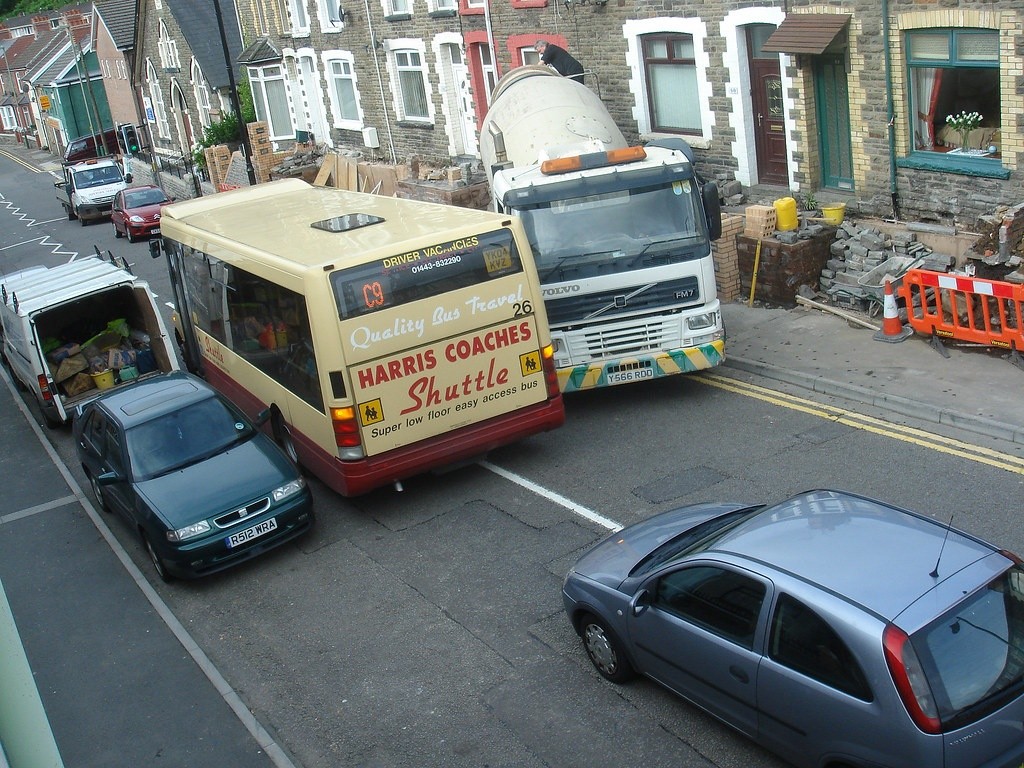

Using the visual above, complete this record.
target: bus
[149,176,567,514]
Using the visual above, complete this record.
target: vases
[960,130,971,152]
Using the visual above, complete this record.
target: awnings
[761,13,852,55]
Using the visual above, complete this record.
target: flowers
[946,111,983,151]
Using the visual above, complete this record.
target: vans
[63,126,119,163]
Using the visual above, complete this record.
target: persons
[534,40,584,86]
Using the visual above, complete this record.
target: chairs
[633,195,676,236]
[146,192,156,198]
[77,175,90,185]
[523,208,561,250]
[125,195,133,203]
[93,170,105,180]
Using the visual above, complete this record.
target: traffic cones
[872,281,914,344]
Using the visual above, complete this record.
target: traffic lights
[122,124,141,156]
[116,128,127,155]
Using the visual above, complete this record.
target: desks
[919,145,1002,159]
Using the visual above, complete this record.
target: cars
[109,184,177,243]
[70,368,319,587]
[560,485,1024,768]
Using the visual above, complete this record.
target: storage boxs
[48,343,81,364]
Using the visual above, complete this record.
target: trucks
[0,250,180,427]
[52,153,134,228]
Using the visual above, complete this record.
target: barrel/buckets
[821,202,846,225]
[90,369,114,390]
[773,197,798,232]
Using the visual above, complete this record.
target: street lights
[57,24,102,158]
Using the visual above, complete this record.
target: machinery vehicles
[475,63,727,393]
